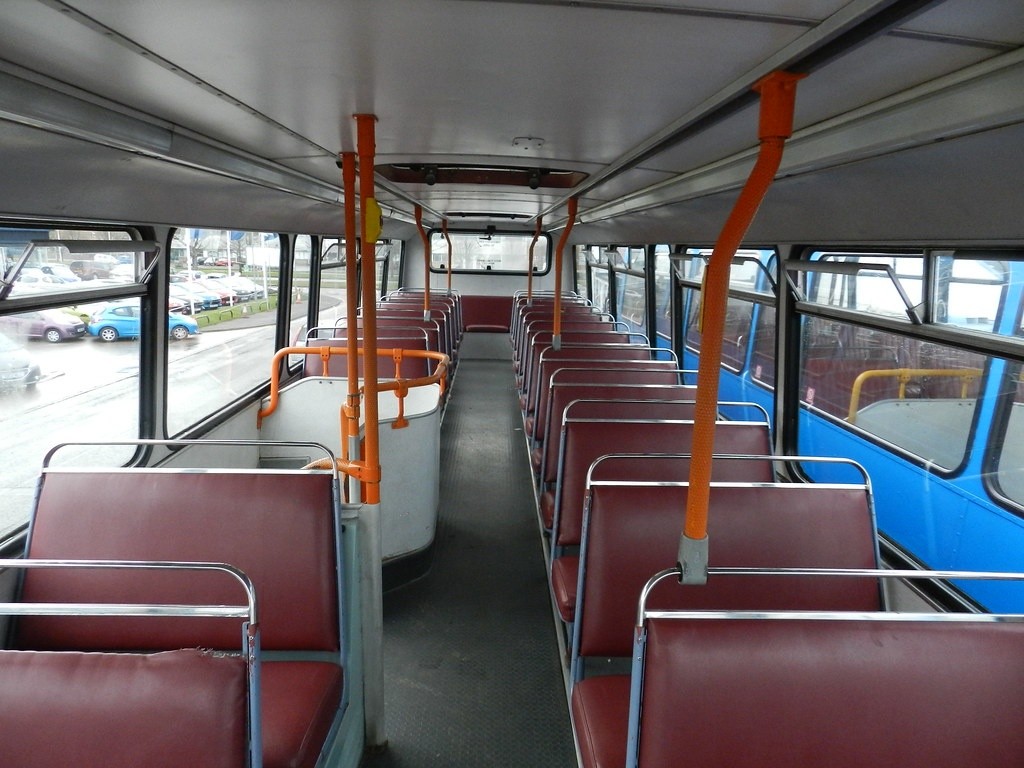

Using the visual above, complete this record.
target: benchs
[0,442,366,767]
[462,294,513,360]
[802,346,900,398]
[303,287,464,378]
[510,289,1024,768]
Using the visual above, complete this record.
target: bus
[594,240,1024,621]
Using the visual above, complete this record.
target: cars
[85,305,199,342]
[3,256,265,314]
[0,308,87,343]
